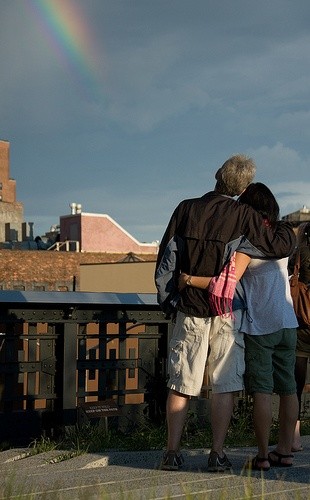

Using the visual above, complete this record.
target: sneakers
[161,450,185,470]
[208,449,233,471]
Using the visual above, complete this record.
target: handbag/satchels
[288,249,310,327]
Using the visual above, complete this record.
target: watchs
[185,275,192,286]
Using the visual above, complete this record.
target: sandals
[245,454,269,472]
[268,450,295,467]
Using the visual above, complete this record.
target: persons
[179,182,297,472]
[285,221,310,452]
[155,155,297,472]
